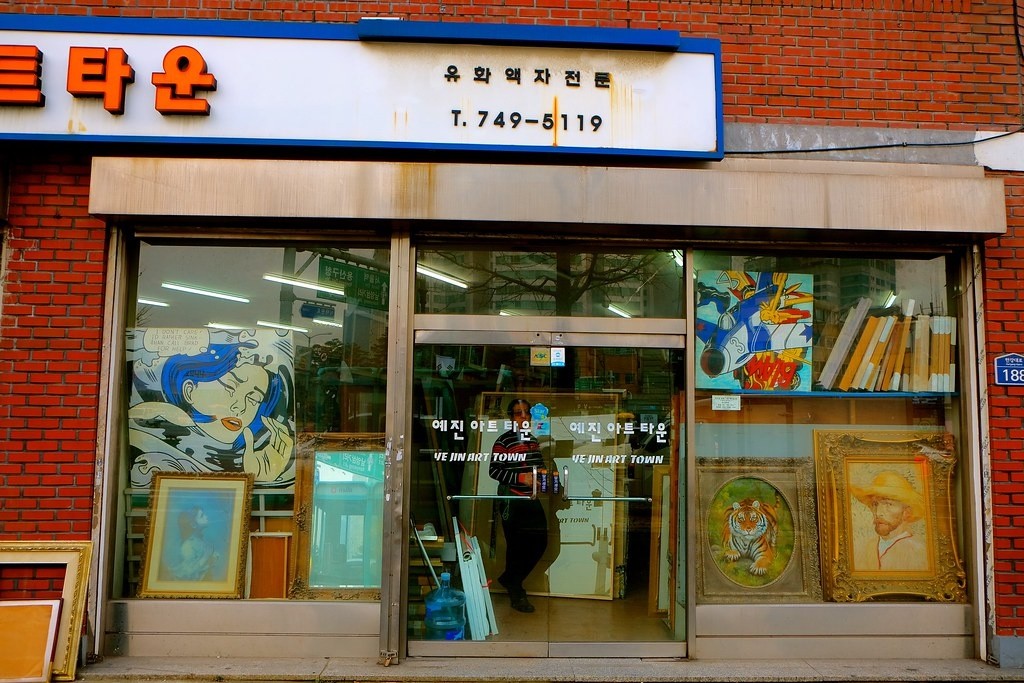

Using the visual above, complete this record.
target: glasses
[513,408,531,416]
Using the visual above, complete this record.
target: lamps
[262,271,345,297]
[137,296,170,307]
[256,320,308,333]
[160,280,250,303]
[668,250,698,279]
[416,263,468,290]
[602,301,632,318]
[203,323,254,330]
[312,318,342,327]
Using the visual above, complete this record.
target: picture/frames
[648,464,672,614]
[0,539,93,682]
[453,516,499,641]
[138,471,254,599]
[342,384,387,432]
[693,457,823,604]
[288,433,385,601]
[812,425,969,605]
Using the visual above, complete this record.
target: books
[813,297,958,393]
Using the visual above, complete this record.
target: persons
[488,399,550,613]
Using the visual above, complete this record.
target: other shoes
[499,576,524,596]
[510,595,535,613]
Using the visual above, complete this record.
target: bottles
[425,573,465,642]
